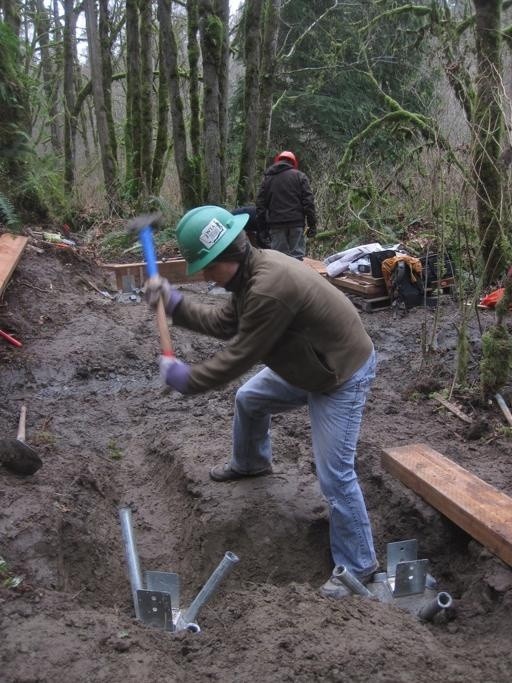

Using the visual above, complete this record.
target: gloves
[157,355,184,385]
[306,226,317,238]
[256,231,268,249]
[141,276,182,315]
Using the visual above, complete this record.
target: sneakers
[320,560,380,600]
[210,462,274,480]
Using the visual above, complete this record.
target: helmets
[175,206,251,277]
[274,151,297,168]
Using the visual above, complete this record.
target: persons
[135,202,381,601]
[260,146,318,261]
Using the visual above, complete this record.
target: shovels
[0,406,43,476]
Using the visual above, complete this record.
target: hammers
[128,214,173,357]
